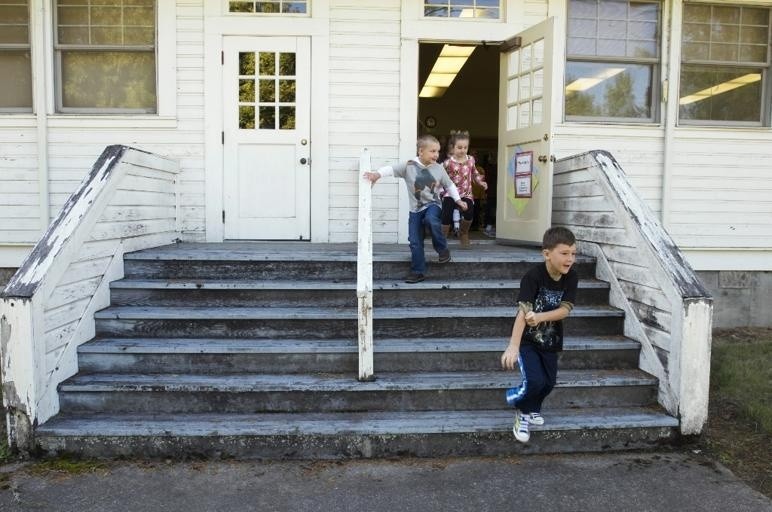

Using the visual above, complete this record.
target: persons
[500,227,579,444]
[440,130,489,249]
[363,136,468,283]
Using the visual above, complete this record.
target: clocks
[425,116,437,128]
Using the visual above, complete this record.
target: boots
[458,220,473,250]
[440,224,451,239]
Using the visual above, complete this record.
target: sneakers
[512,410,545,444]
[438,249,451,264]
[405,274,425,283]
